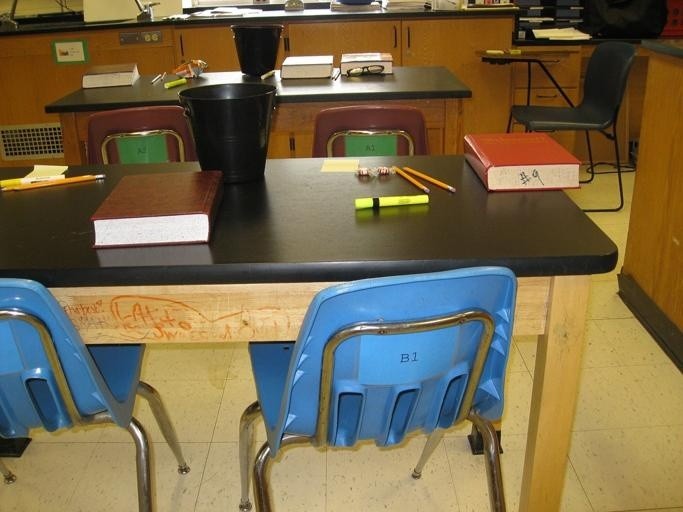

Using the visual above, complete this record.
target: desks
[46,66,474,164]
[1,155,619,512]
[475,44,580,65]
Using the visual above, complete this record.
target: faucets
[133,0,161,21]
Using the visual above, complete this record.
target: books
[532,27,593,41]
[329,1,381,13]
[463,133,582,194]
[91,170,221,247]
[341,52,393,76]
[281,55,335,78]
[193,7,264,17]
[386,0,425,13]
[81,64,141,89]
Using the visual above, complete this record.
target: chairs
[85,106,197,164]
[239,265,518,512]
[311,106,430,158]
[1,279,191,512]
[506,40,639,212]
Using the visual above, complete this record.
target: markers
[164,78,187,89]
[353,195,432,210]
[261,70,275,80]
[0,174,65,187]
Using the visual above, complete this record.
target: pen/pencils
[332,71,340,80]
[0,174,106,192]
[335,72,342,80]
[151,74,161,84]
[160,72,167,81]
[392,164,430,194]
[403,166,456,194]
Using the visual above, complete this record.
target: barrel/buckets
[176,83,279,186]
[231,24,284,78]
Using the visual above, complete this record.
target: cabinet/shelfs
[174,15,519,163]
[509,34,578,154]
